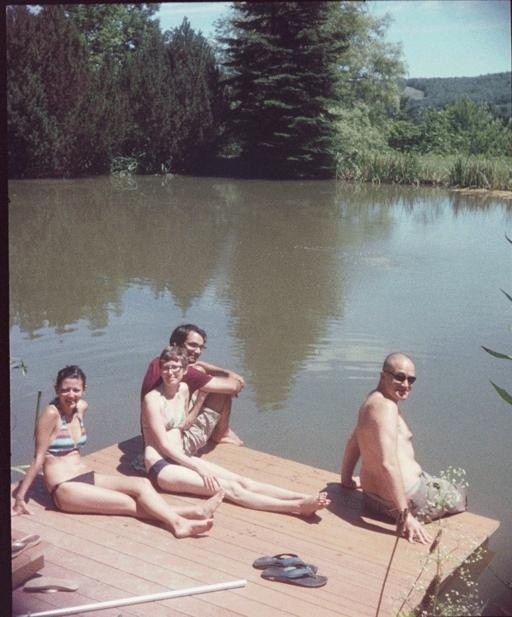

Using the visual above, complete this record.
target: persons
[140,347,333,519]
[11,365,225,540]
[341,352,468,545]
[138,324,245,447]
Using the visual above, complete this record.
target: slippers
[23,576,80,594]
[253,553,328,588]
[11,533,41,559]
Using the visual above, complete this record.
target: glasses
[384,370,416,385]
[160,365,184,373]
